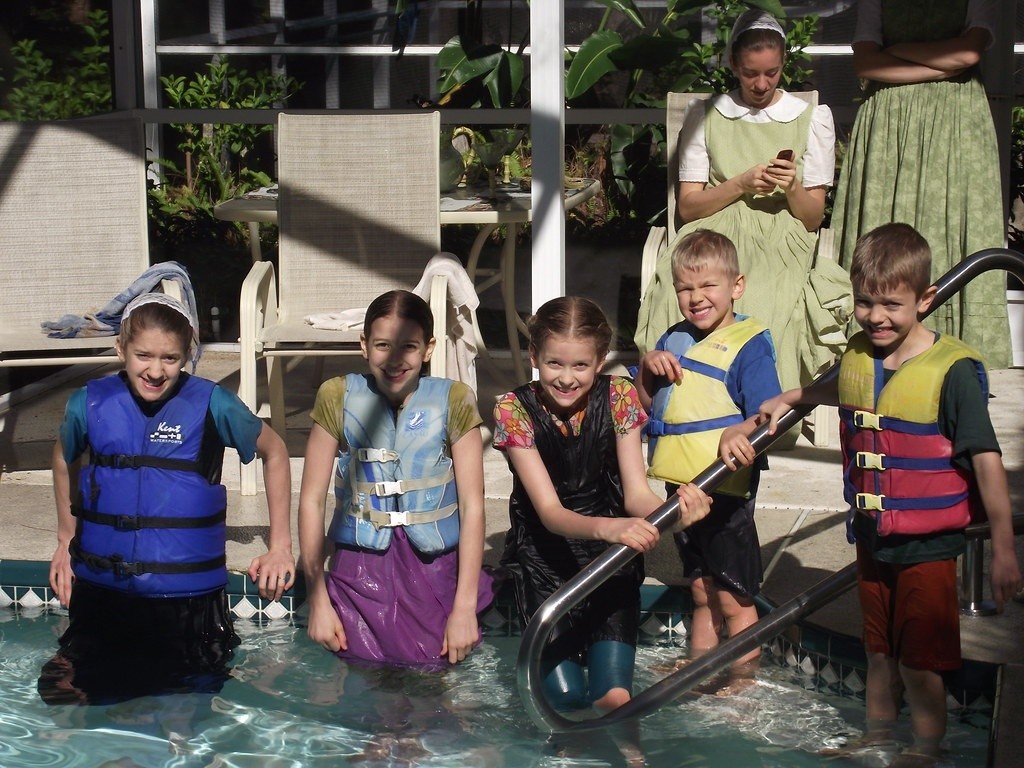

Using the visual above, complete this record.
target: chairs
[0,118,195,377]
[639,89,836,447]
[238,111,449,497]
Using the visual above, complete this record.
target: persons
[298,291,507,665]
[759,223,1022,737]
[633,10,835,450]
[493,297,713,717]
[50,293,296,703]
[830,1,1013,369]
[635,230,783,650]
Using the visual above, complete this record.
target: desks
[213,176,602,395]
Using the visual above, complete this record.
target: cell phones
[774,149,793,168]
